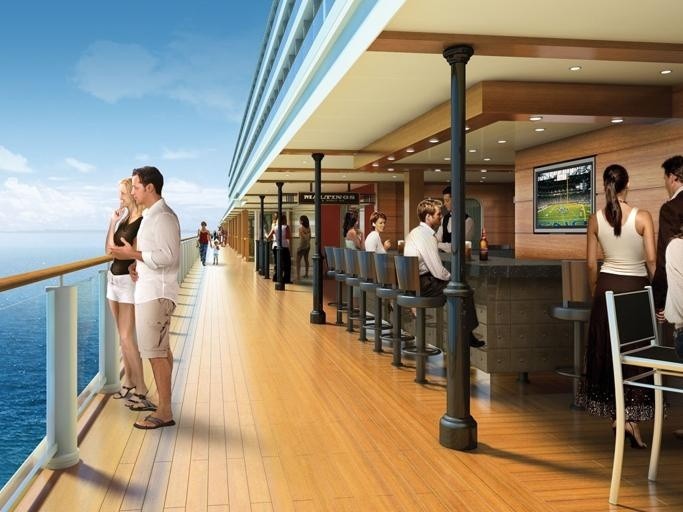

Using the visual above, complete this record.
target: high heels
[621,414,650,451]
[111,384,149,406]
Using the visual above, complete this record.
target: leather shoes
[467,331,486,350]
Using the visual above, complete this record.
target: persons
[209,241,219,266]
[268,215,292,284]
[209,227,228,248]
[196,220,211,266]
[402,198,486,348]
[582,164,669,450]
[649,155,683,325]
[295,214,311,280]
[341,207,360,250]
[107,166,179,428]
[106,178,148,408]
[662,228,683,364]
[435,186,473,253]
[363,211,387,254]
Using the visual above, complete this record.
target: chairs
[545,256,601,414]
[322,243,448,386]
[604,283,682,507]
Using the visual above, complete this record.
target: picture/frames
[529,153,598,237]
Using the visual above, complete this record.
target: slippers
[130,414,178,430]
[129,399,161,413]
